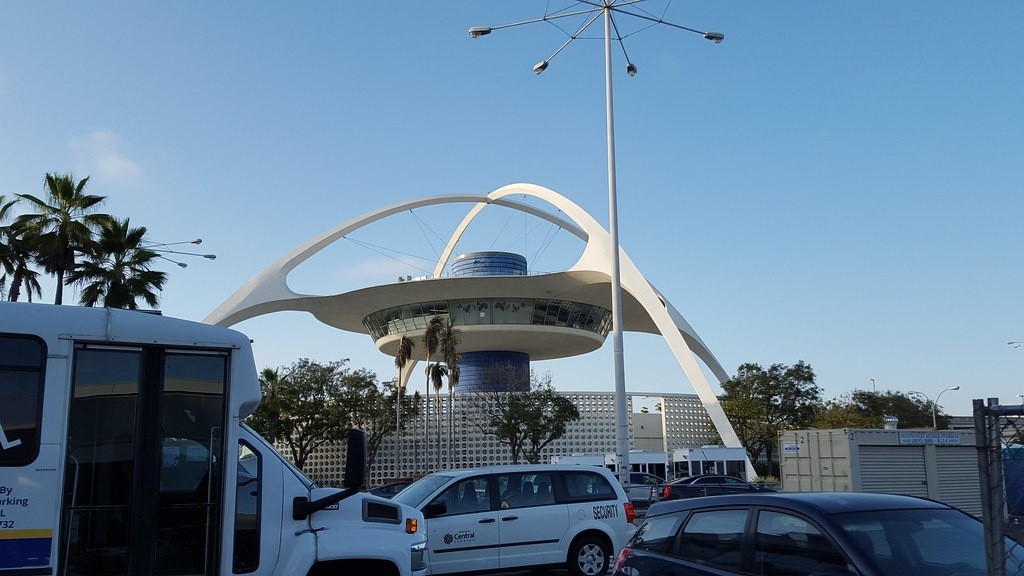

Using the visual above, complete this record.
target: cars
[523,473,593,493]
[650,474,780,502]
[366,480,489,510]
[612,490,1024,576]
[389,463,635,576]
[587,471,667,502]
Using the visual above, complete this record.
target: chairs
[537,481,549,499]
[159,465,187,490]
[522,482,534,503]
[457,483,478,513]
[502,475,521,503]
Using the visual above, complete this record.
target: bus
[1,300,428,576]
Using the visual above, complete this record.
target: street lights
[1017,394,1024,402]
[131,239,217,278]
[908,386,960,427]
[467,8,725,497]
[869,379,875,393]
